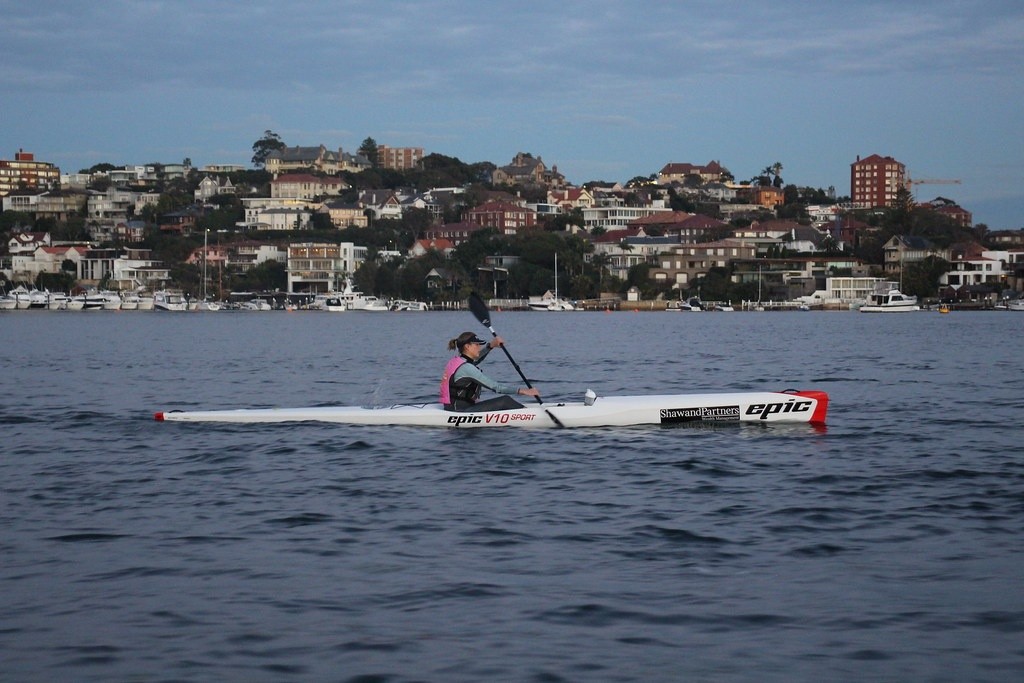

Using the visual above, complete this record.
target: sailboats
[754,264,765,311]
[528,252,576,312]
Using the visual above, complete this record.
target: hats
[457,334,486,348]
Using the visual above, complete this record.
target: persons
[440,332,540,412]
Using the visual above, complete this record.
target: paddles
[467,291,564,427]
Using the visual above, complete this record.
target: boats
[154,388,831,428]
[940,303,950,313]
[858,289,920,312]
[0,229,271,311]
[993,299,1024,311]
[665,295,734,312]
[315,291,428,312]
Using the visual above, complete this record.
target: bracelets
[518,388,520,394]
